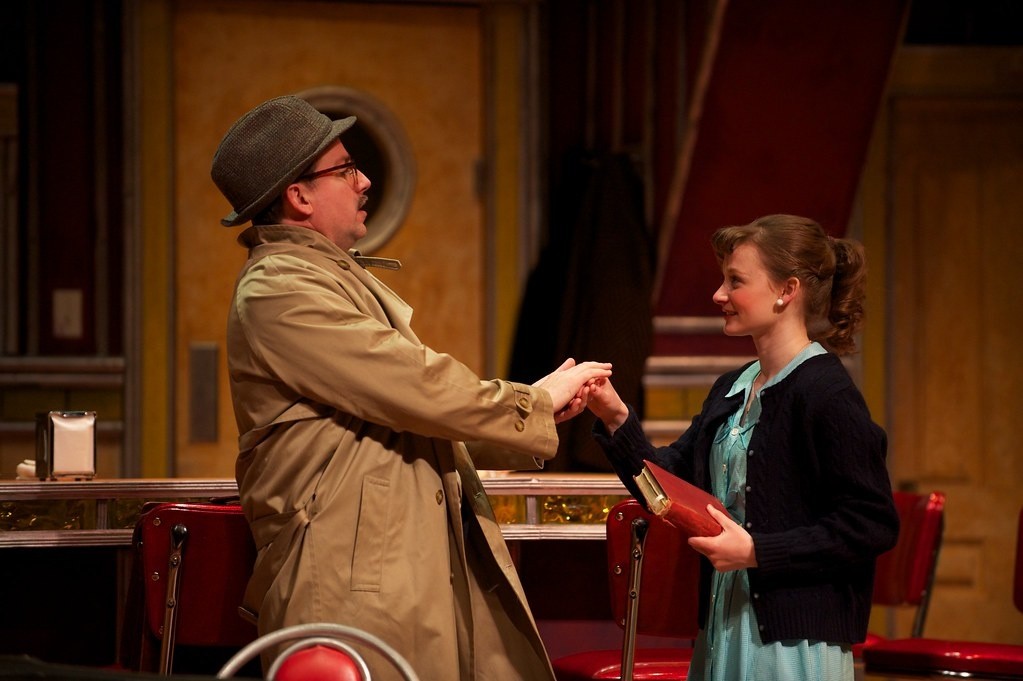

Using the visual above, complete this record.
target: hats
[210,94,357,226]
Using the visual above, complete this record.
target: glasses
[294,160,359,188]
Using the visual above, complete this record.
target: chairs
[551,498,711,681]
[861,509,1023,680]
[217,625,419,681]
[846,491,946,661]
[131,500,260,675]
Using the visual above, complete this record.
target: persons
[208,98,614,681]
[586,215,902,680]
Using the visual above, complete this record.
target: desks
[0,474,630,681]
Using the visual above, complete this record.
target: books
[632,459,732,538]
